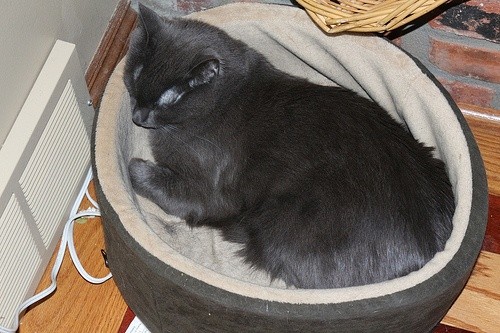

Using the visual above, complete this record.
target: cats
[124,3,454,290]
